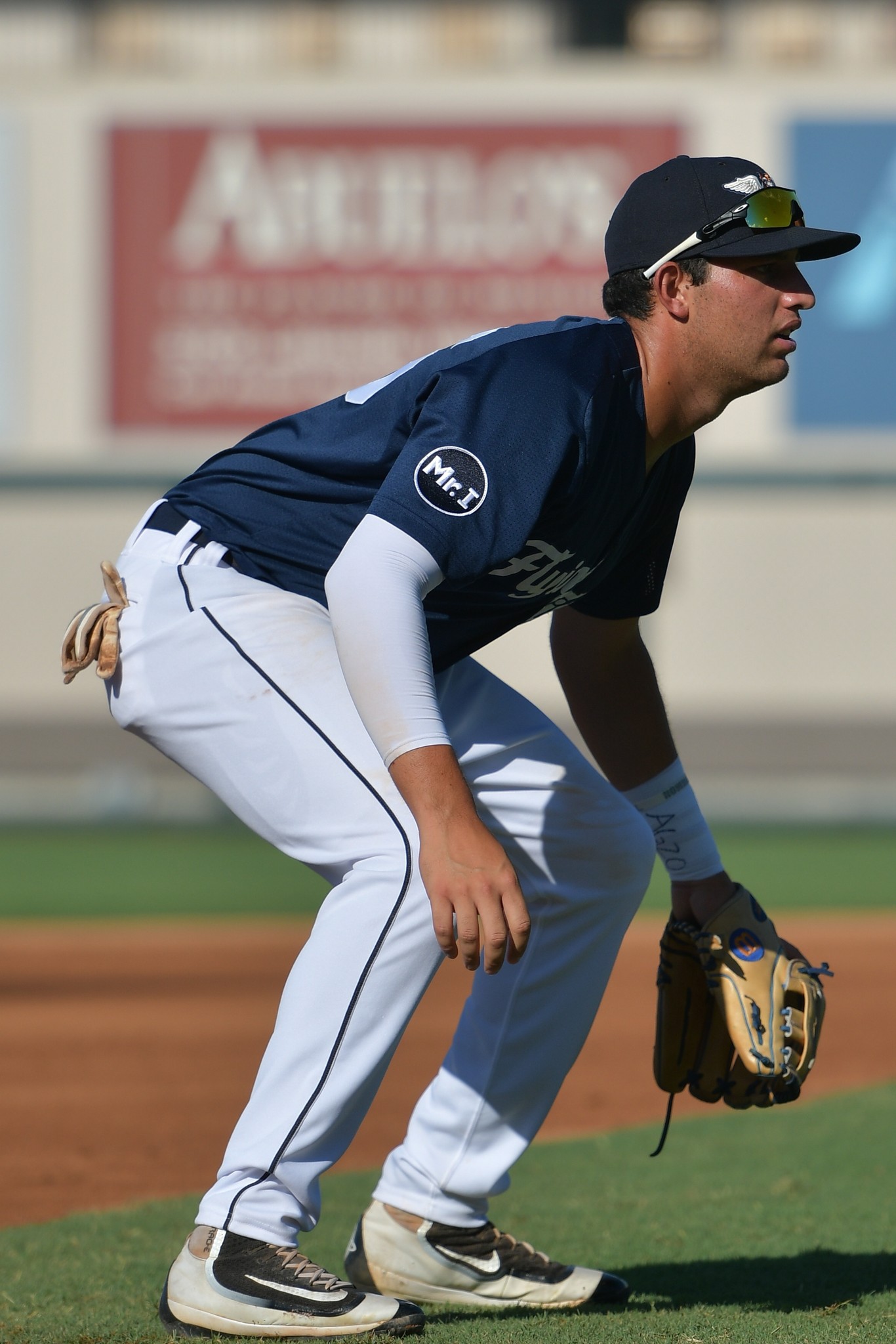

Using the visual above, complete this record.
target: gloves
[58,565,133,687]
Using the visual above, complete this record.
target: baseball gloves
[653,882,825,1111]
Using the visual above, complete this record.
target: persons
[93,153,861,1340]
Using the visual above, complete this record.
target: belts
[147,497,233,563]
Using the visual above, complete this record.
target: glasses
[644,186,806,280]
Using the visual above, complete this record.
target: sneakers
[158,1227,426,1341]
[344,1198,631,1312]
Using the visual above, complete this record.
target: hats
[605,154,861,278]
